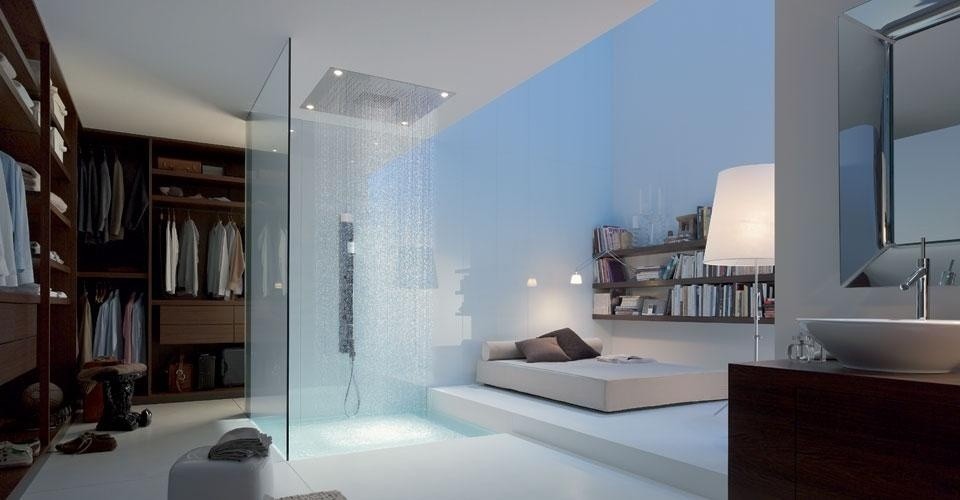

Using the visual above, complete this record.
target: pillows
[480,337,603,361]
[537,327,602,362]
[513,336,571,364]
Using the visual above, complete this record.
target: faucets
[900,237,933,319]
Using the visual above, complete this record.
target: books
[635,264,669,281]
[673,254,773,278]
[593,291,668,317]
[593,225,633,255]
[664,256,675,281]
[670,283,776,317]
[598,257,625,282]
[665,205,711,242]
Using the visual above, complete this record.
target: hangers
[77,276,148,304]
[153,201,245,230]
[85,145,124,162]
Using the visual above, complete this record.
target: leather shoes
[26,416,61,431]
[55,431,117,454]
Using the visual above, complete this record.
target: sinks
[794,317,960,373]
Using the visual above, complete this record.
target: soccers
[22,382,63,416]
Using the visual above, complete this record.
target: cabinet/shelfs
[45,43,79,447]
[592,230,776,325]
[150,136,246,396]
[76,120,152,396]
[0,0,46,453]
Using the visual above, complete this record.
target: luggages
[165,346,193,393]
[222,347,244,387]
[193,345,216,390]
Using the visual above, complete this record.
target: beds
[475,354,728,415]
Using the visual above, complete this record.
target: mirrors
[883,12,960,245]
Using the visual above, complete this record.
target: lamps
[702,164,779,362]
[525,276,538,288]
[570,249,635,285]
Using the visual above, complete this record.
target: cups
[789,334,815,364]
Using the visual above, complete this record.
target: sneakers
[0,441,40,468]
[96,408,153,431]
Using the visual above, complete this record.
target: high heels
[50,407,69,422]
